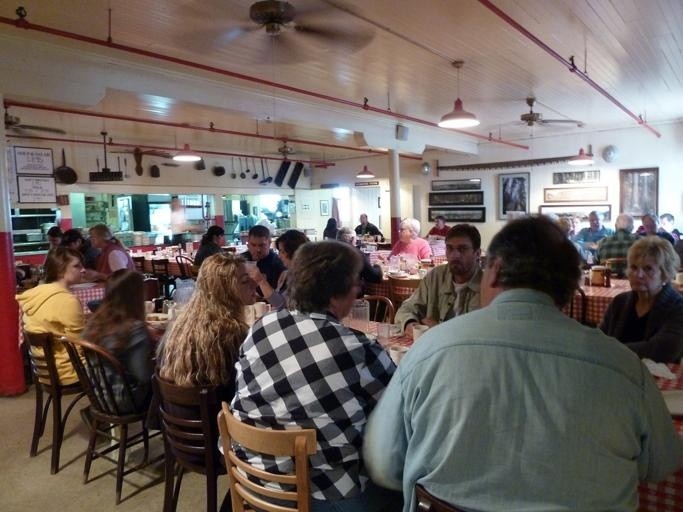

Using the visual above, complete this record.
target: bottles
[163,299,176,321]
[583,264,613,288]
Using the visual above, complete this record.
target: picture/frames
[539,170,611,226]
[428,178,486,223]
[498,172,529,221]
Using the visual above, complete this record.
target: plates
[661,390,683,418]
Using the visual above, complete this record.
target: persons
[15,193,683,512]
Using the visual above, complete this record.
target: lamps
[566,147,594,165]
[421,162,431,177]
[173,143,201,162]
[438,60,480,130]
[4,101,66,143]
[356,164,377,179]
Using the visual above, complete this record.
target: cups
[377,325,390,346]
[347,298,371,335]
[175,279,195,313]
[385,253,417,278]
[412,325,428,343]
[389,345,408,365]
[254,301,272,319]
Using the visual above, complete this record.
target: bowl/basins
[146,313,170,329]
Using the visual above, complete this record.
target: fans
[173,0,377,68]
[491,97,586,130]
